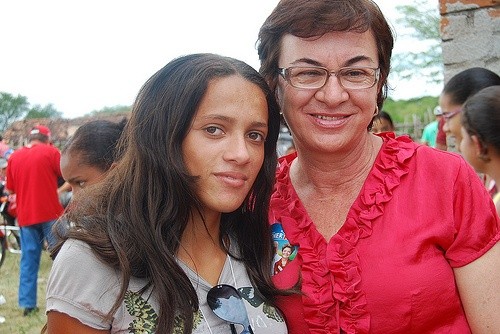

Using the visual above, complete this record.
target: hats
[30,125,51,136]
[434,106,443,115]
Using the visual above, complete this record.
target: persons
[273,239,295,276]
[6,125,67,316]
[370,67,500,220]
[0,135,73,250]
[45,53,306,334]
[59,118,127,202]
[255,0,500,334]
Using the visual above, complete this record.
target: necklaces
[178,258,209,282]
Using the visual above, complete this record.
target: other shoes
[24,306,40,315]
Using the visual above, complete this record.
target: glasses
[442,110,461,121]
[207,283,255,334]
[277,66,380,89]
